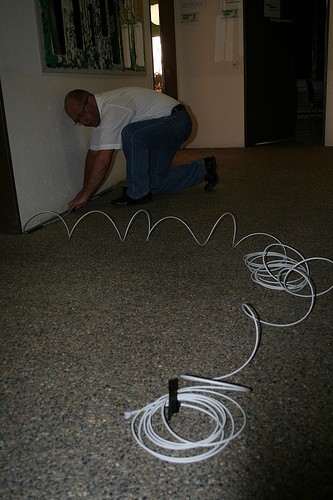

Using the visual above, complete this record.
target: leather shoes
[204,155,220,194]
[108,192,154,208]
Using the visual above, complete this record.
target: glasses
[73,96,88,126]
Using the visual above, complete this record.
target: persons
[62,86,220,211]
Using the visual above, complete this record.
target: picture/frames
[33,0,148,76]
